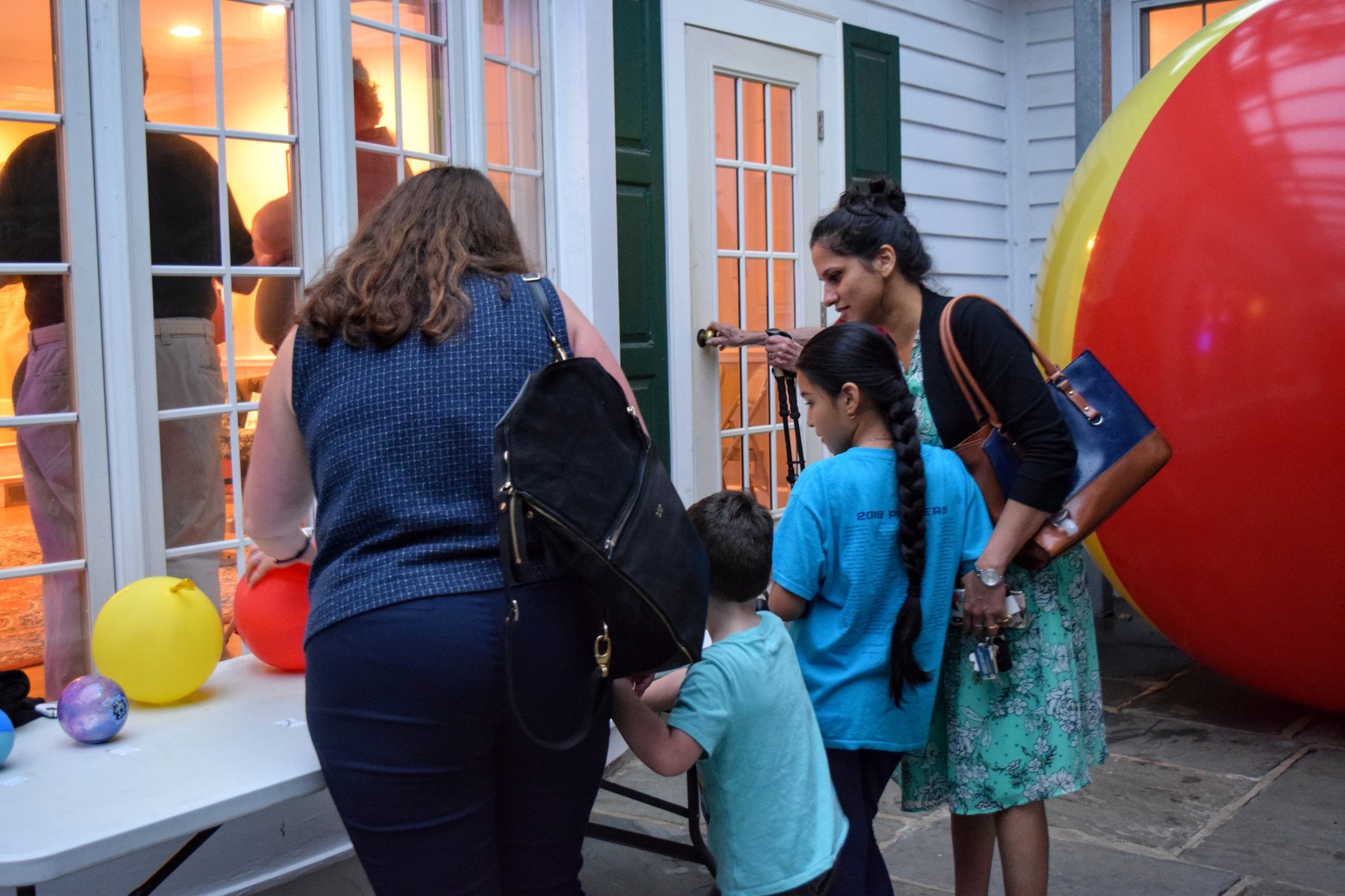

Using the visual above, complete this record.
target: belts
[28,318,217,353]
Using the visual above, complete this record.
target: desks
[1,639,715,896]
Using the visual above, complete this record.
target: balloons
[92,576,223,704]
[234,542,317,674]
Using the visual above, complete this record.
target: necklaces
[855,438,893,447]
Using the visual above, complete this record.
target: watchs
[973,561,1004,587]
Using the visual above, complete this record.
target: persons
[251,58,414,355]
[0,47,258,702]
[241,167,649,896]
[704,317,845,372]
[767,320,993,896]
[809,175,1109,896]
[611,490,850,896]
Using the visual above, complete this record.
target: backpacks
[492,271,709,750]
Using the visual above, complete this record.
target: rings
[769,352,771,357]
[988,624,997,629]
[253,554,260,564]
[975,625,983,627]
[633,678,636,690]
[1002,619,1009,622]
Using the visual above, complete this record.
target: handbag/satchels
[939,293,1172,571]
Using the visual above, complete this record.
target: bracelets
[273,536,312,564]
